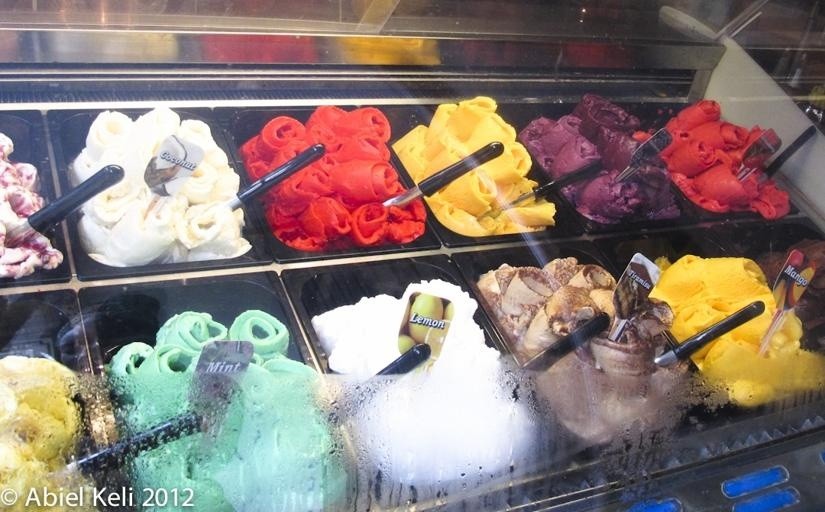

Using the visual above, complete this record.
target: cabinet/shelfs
[1,1,825,512]
